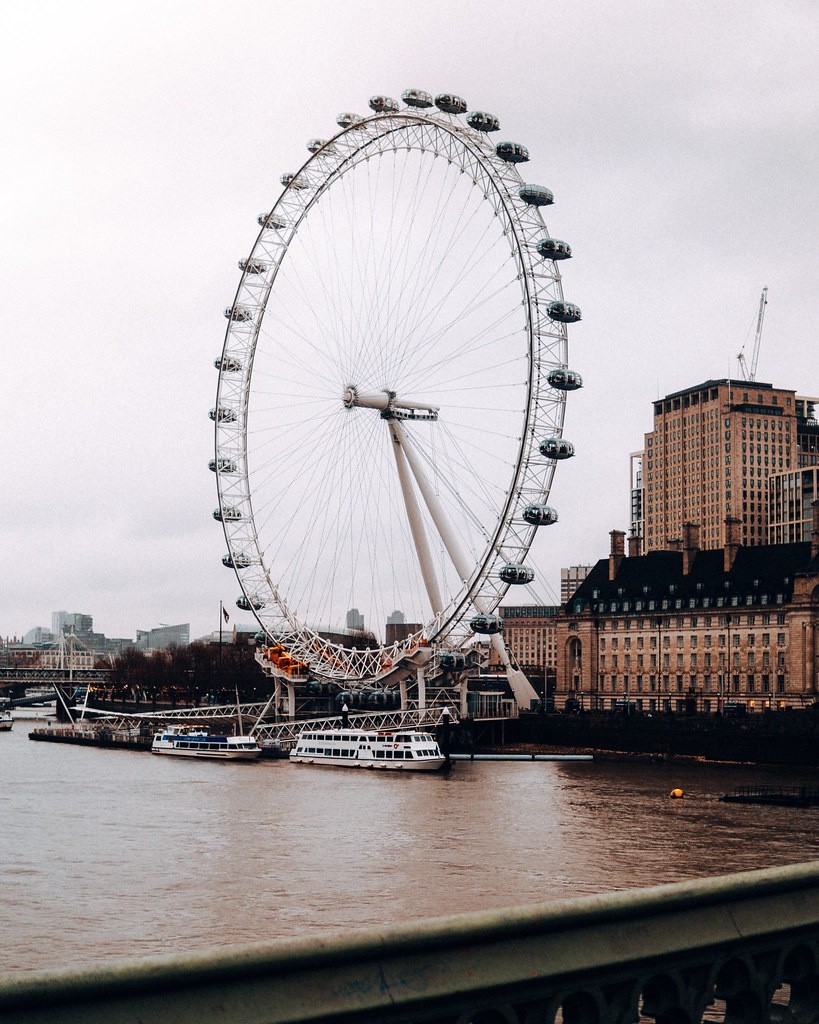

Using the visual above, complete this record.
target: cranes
[737,284,769,380]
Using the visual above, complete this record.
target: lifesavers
[393,743,399,750]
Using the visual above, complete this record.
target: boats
[152,725,263,759]
[0,720,13,730]
[290,729,446,770]
